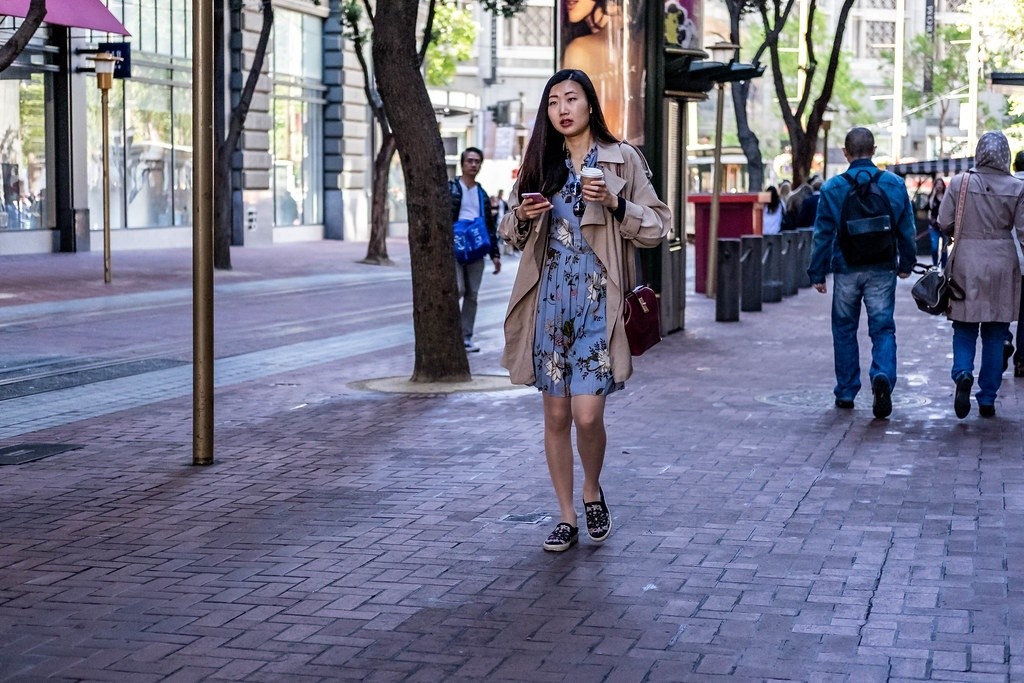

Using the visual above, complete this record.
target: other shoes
[1014,362,1024,378]
[871,375,892,418]
[954,373,974,420]
[835,399,855,409]
[465,339,481,353]
[1003,340,1015,372]
[979,405,996,418]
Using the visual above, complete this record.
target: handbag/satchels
[623,284,663,356]
[452,216,492,265]
[913,263,966,315]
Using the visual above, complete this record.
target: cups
[580,167,604,203]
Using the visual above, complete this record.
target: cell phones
[521,192,551,208]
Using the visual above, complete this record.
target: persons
[779,181,792,206]
[926,178,953,269]
[807,129,918,419]
[783,184,815,232]
[938,129,1024,421]
[1003,151,1024,378]
[762,186,787,235]
[807,175,819,186]
[812,181,823,218]
[446,147,501,356]
[495,67,671,554]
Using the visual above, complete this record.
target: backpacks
[841,170,898,266]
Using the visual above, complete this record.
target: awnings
[0,0,131,37]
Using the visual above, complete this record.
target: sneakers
[583,484,612,543]
[543,522,579,552]
